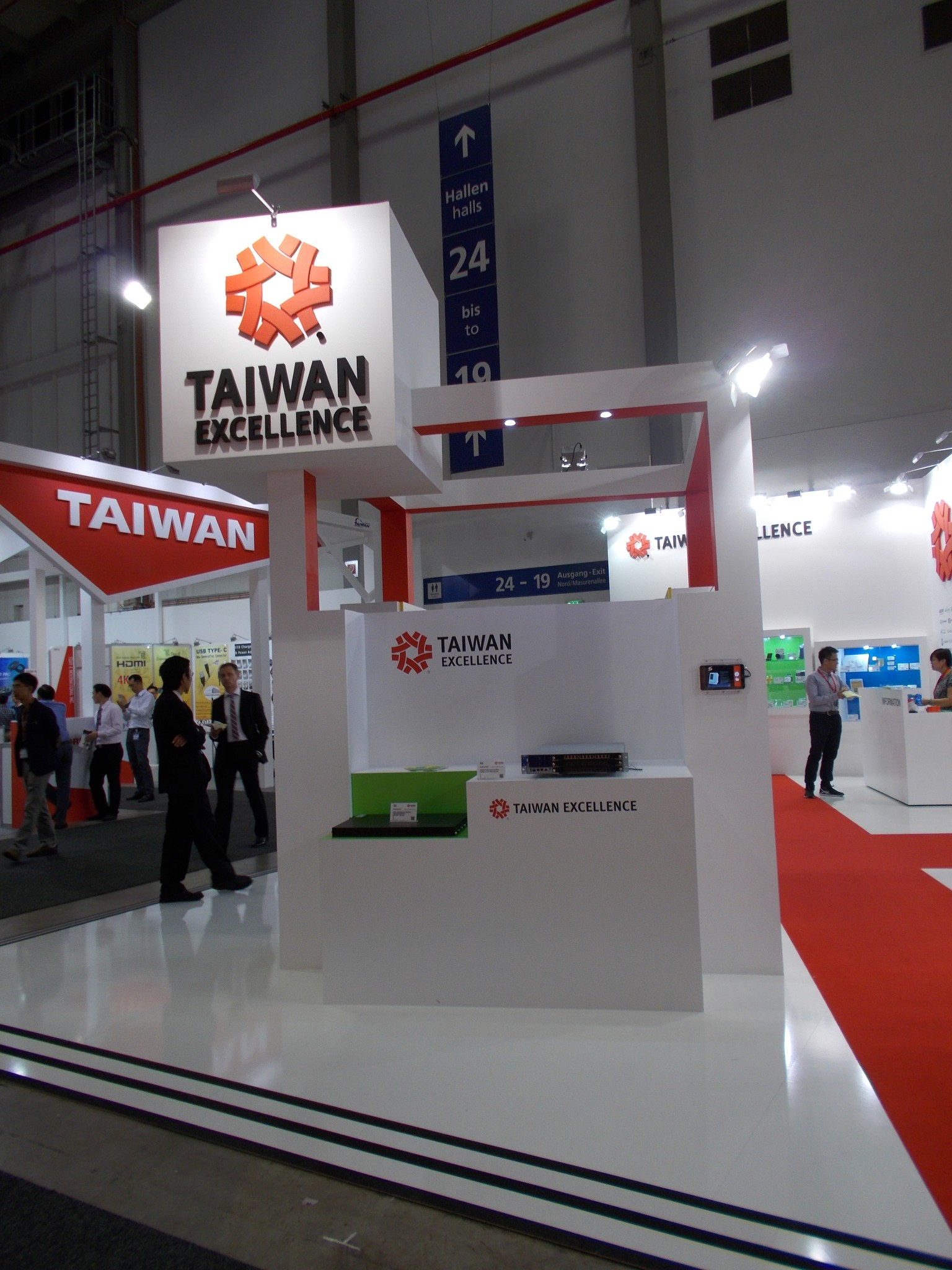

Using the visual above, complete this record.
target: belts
[810,711,839,716]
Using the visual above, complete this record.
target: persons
[153,654,256,903]
[116,674,157,805]
[919,647,952,711]
[9,692,22,712]
[83,683,124,822]
[0,673,60,865]
[36,682,73,832]
[146,685,159,703]
[208,661,270,855]
[0,692,18,731]
[803,646,855,799]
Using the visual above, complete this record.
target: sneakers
[804,786,815,798]
[819,784,844,797]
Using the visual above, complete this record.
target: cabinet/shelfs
[858,687,952,805]
[0,715,99,828]
[762,657,808,708]
[833,668,920,722]
[120,719,218,790]
[318,765,703,1016]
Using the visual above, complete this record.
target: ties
[228,694,239,740]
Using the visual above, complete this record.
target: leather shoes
[26,844,59,858]
[52,789,156,830]
[3,847,21,861]
[251,836,269,848]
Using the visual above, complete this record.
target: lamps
[159,633,251,647]
[713,334,790,408]
[0,646,14,655]
[600,516,622,534]
[560,442,588,473]
[829,431,951,502]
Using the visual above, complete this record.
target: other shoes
[159,883,204,903]
[212,871,252,890]
